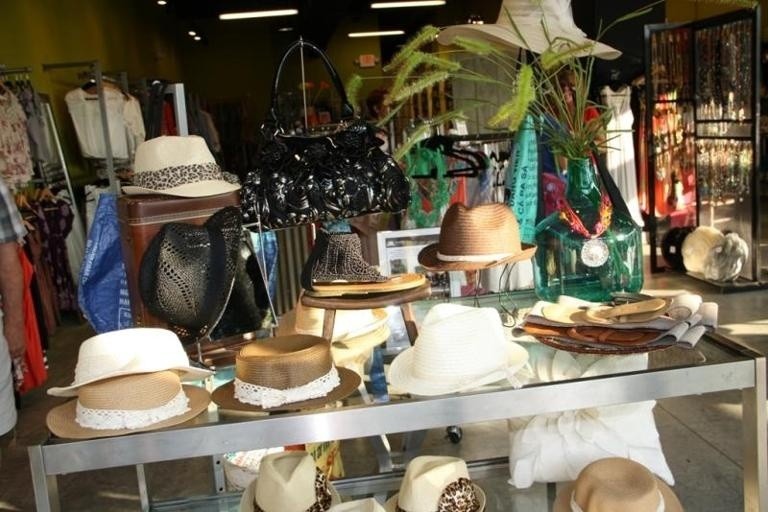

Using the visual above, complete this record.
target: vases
[533,158,647,306]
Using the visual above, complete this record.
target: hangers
[17,184,70,221]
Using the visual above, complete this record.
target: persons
[549,70,606,177]
[368,88,407,153]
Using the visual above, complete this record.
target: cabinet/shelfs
[25,330,768,511]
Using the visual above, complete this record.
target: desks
[301,281,432,353]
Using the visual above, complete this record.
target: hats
[122,133,242,197]
[273,286,395,361]
[554,457,685,512]
[388,302,529,396]
[138,206,241,346]
[438,0,622,60]
[212,334,360,411]
[383,455,486,512]
[326,499,387,512]
[418,202,538,271]
[240,449,342,512]
[47,327,211,439]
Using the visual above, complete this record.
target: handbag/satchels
[239,122,412,232]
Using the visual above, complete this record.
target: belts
[511,296,719,354]
